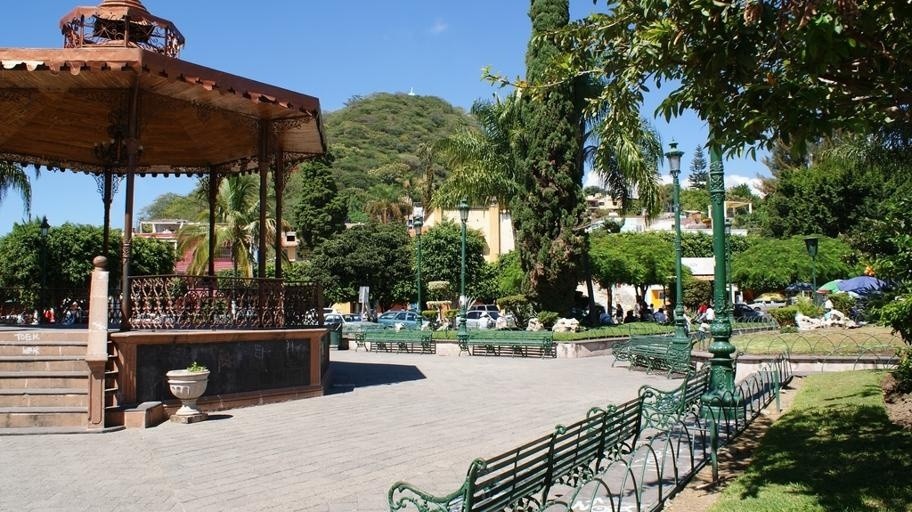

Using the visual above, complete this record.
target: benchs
[467,329,555,359]
[355,330,433,354]
[611,332,694,379]
[638,352,743,438]
[389,389,654,512]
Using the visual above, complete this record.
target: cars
[296,303,505,333]
[4,298,180,330]
[730,300,785,324]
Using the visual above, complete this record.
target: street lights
[805,235,819,303]
[39,215,51,310]
[456,195,472,338]
[660,138,691,340]
[411,213,427,325]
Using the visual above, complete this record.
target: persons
[478,311,490,329]
[849,308,859,321]
[599,299,715,324]
[825,297,833,309]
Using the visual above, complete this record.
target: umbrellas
[785,283,815,292]
[815,280,840,294]
[837,275,888,295]
[754,292,788,301]
